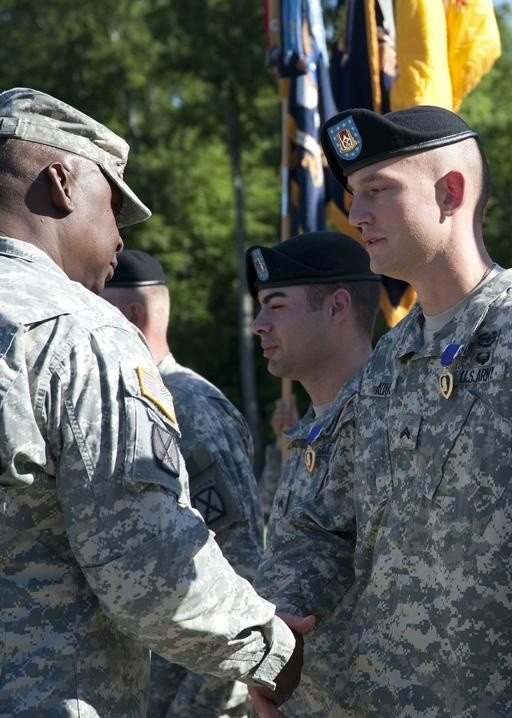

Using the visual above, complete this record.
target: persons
[98,245,267,716]
[254,103,512,717]
[245,229,383,525]
[1,82,321,717]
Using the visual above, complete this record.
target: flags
[257,0,504,329]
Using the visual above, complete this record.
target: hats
[104,250,167,288]
[246,231,382,304]
[0,87,152,228]
[320,106,480,195]
